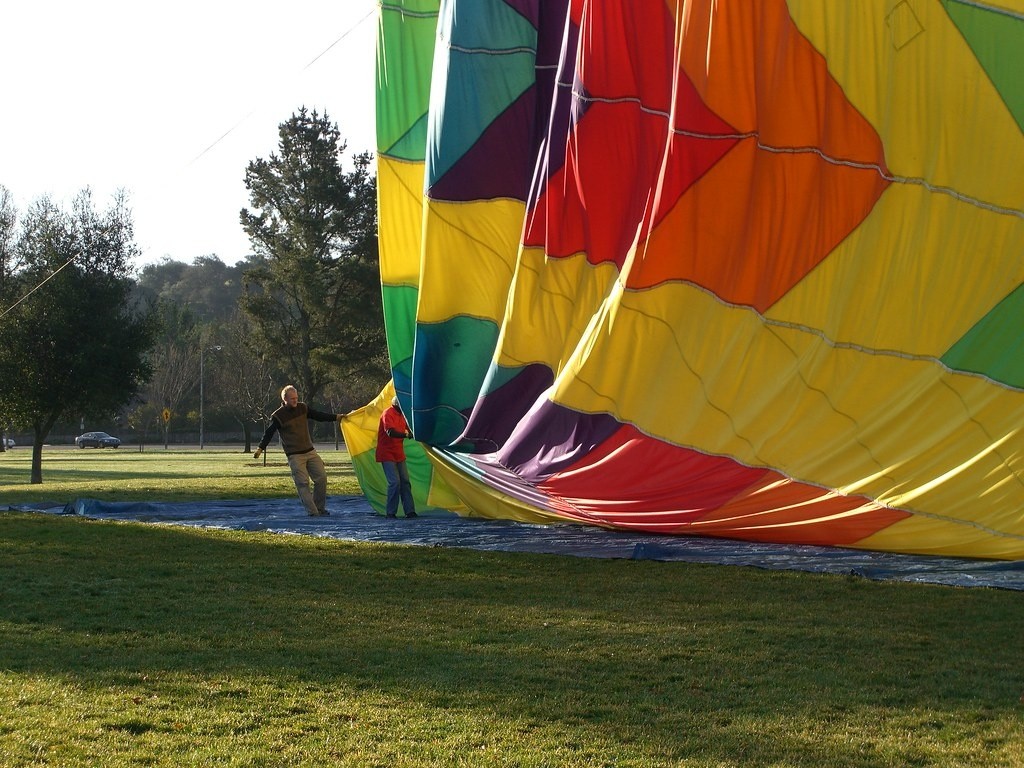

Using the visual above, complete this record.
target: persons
[253,385,348,518]
[375,395,419,520]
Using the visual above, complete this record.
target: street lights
[200,345,220,450]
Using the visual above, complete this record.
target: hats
[392,396,399,406]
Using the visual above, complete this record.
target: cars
[2,435,16,449]
[75,431,121,449]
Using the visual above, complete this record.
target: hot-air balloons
[338,1,1024,566]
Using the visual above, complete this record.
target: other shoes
[406,512,417,518]
[319,510,330,516]
[387,514,396,519]
